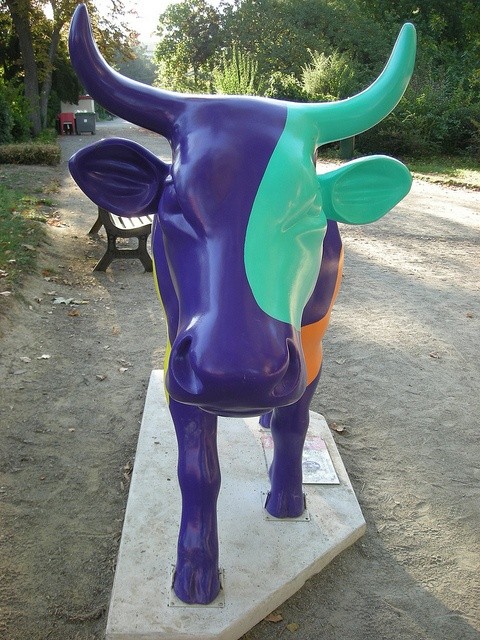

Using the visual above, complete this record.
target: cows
[65,1,418,607]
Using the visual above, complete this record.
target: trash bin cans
[75,111,96,135]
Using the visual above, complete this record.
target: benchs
[86,206,154,275]
